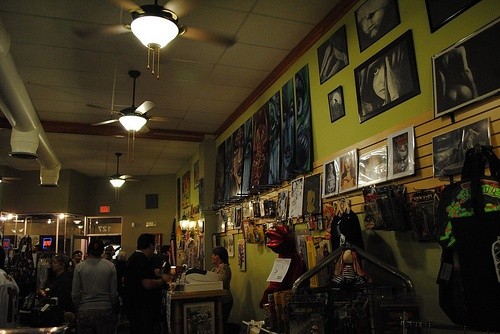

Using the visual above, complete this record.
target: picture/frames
[354,0,401,53]
[316,25,350,84]
[327,85,345,124]
[353,29,421,123]
[431,18,500,119]
[425,0,481,33]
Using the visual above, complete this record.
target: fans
[73,0,238,49]
[86,70,185,132]
[85,153,142,183]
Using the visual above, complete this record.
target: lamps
[117,105,149,134]
[128,0,187,81]
[108,174,126,188]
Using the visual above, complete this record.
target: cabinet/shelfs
[170,289,228,334]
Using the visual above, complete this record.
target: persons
[72,239,123,334]
[209,246,233,332]
[40,246,115,325]
[123,233,171,334]
[0,246,22,329]
[437,45,478,113]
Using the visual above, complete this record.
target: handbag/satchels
[18,292,62,328]
